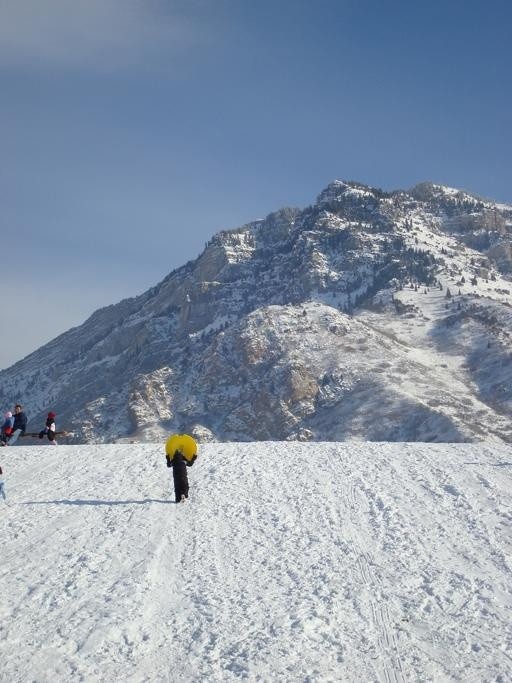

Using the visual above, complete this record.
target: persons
[0,466,7,501]
[0,405,27,447]
[39,412,57,446]
[166,449,198,503]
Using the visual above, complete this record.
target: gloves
[192,455,197,460]
[166,455,170,459]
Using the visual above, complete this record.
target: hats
[48,412,56,418]
[4,412,12,418]
